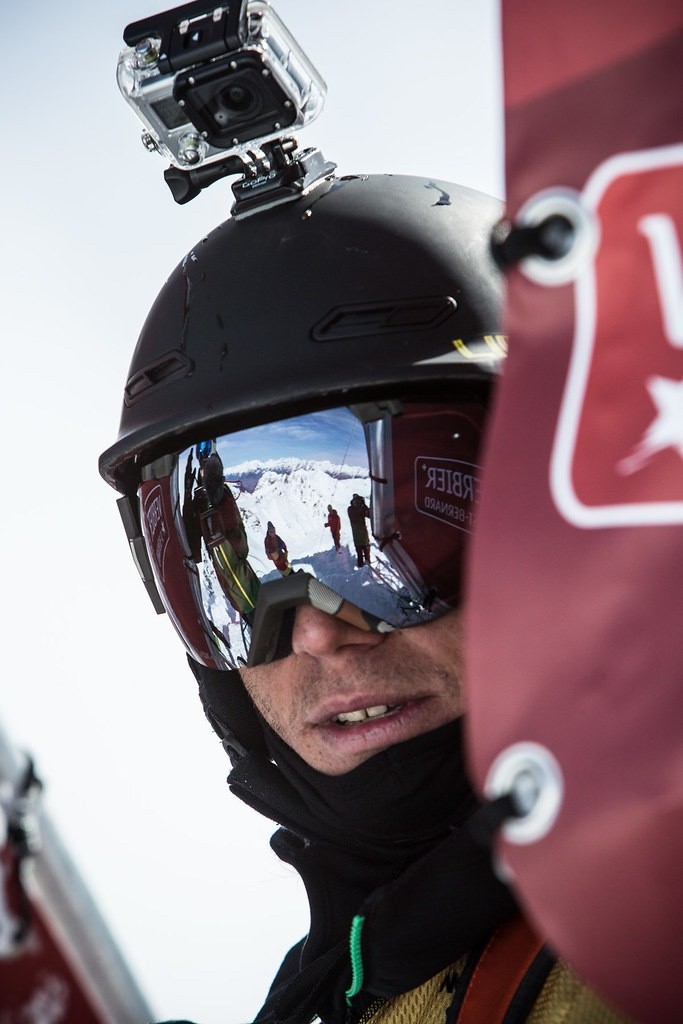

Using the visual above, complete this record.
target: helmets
[96,168,526,842]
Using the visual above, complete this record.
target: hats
[267,520,274,530]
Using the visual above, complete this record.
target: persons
[265,521,295,577]
[325,505,342,553]
[98,173,620,1024]
[183,438,261,629]
[348,493,371,568]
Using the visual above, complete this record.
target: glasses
[123,393,495,670]
